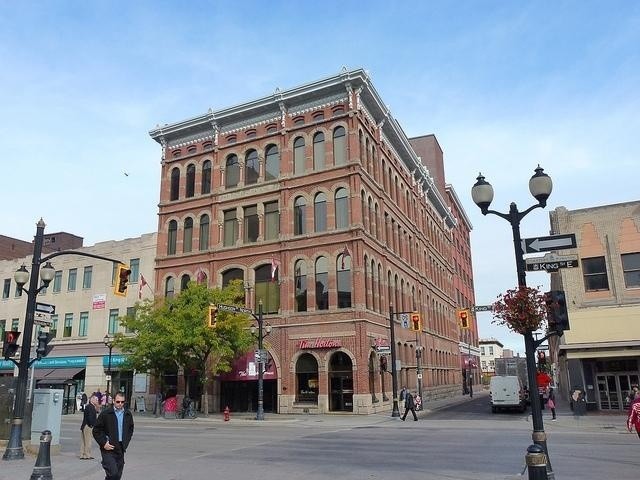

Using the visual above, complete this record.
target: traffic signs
[521,234,578,271]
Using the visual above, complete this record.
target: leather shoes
[80,458,84,460]
[85,457,95,459]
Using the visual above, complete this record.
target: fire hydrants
[224,406,230,421]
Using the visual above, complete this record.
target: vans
[489,375,528,414]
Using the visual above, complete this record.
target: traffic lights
[37,332,55,357]
[412,312,421,333]
[208,306,218,328]
[3,332,20,356]
[114,263,132,297]
[539,351,546,365]
[457,308,471,329]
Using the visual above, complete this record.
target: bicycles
[176,401,198,420]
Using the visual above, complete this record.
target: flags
[342,245,350,270]
[271,256,278,282]
[197,270,206,284]
[138,275,147,299]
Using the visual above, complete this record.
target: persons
[625,391,640,439]
[399,389,418,421]
[399,386,406,409]
[631,384,640,400]
[544,387,556,421]
[79,396,99,460]
[78,389,114,413]
[412,391,422,411]
[91,391,135,479]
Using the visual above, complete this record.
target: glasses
[116,401,124,403]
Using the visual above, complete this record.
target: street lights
[103,333,119,408]
[470,165,558,480]
[250,321,272,418]
[2,259,55,461]
[415,346,424,413]
